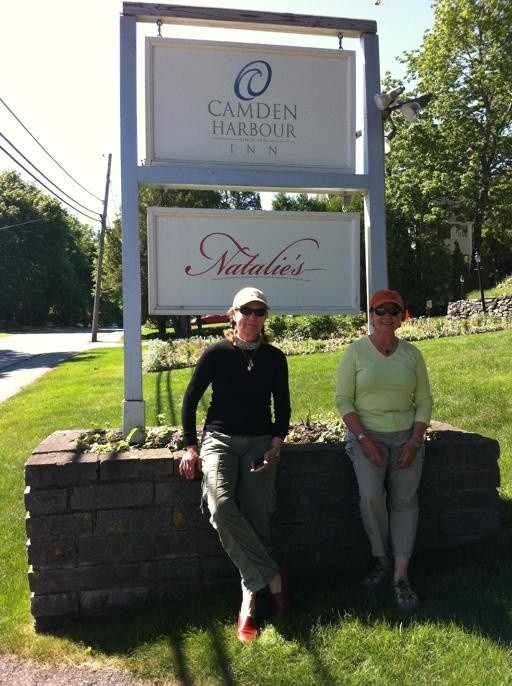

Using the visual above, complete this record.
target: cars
[190,315,230,326]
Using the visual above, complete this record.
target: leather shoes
[270,567,288,614]
[237,612,259,644]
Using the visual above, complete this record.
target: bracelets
[357,432,369,440]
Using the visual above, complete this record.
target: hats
[371,289,404,309]
[233,287,269,310]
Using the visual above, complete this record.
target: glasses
[375,306,400,316]
[235,307,267,318]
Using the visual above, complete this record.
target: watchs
[409,436,422,450]
[272,444,283,464]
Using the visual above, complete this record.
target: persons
[335,291,434,616]
[179,287,292,644]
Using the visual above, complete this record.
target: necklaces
[240,348,259,372]
[369,335,397,353]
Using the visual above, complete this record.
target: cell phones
[254,455,265,472]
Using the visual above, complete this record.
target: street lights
[474,250,486,314]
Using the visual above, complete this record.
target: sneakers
[393,579,418,611]
[359,563,394,586]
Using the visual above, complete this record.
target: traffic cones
[405,308,411,320]
